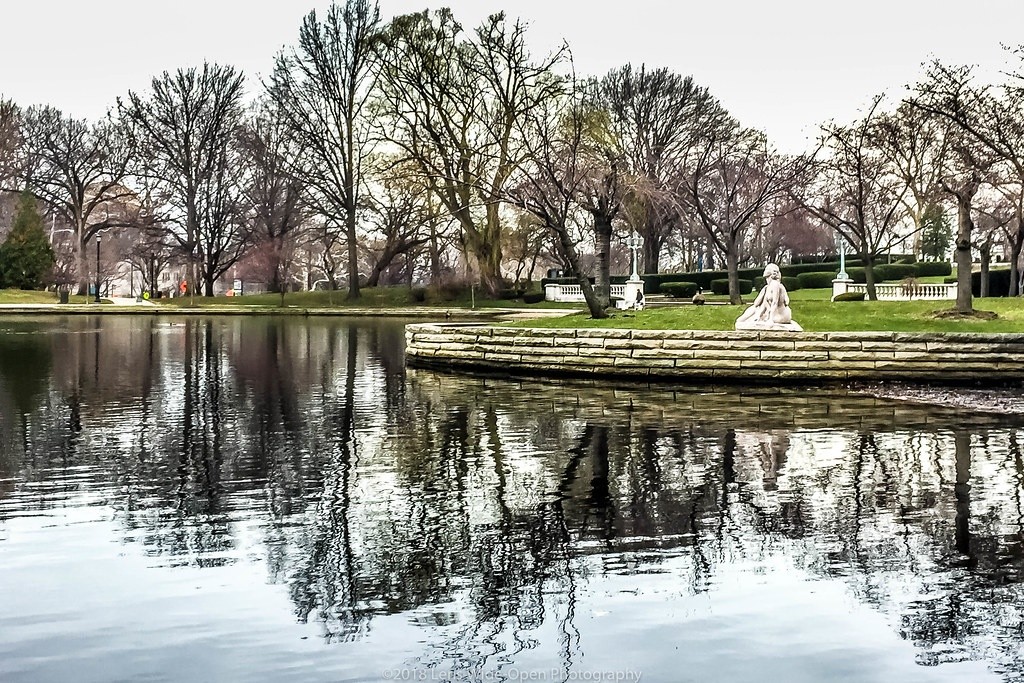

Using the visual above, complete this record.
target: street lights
[149,249,155,299]
[624,230,646,284]
[94,233,102,303]
[831,223,856,283]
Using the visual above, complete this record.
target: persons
[735,262,805,331]
[697,254,702,272]
[179,278,189,298]
[445,311,452,319]
[636,289,644,304]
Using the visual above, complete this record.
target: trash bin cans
[60,290,69,303]
